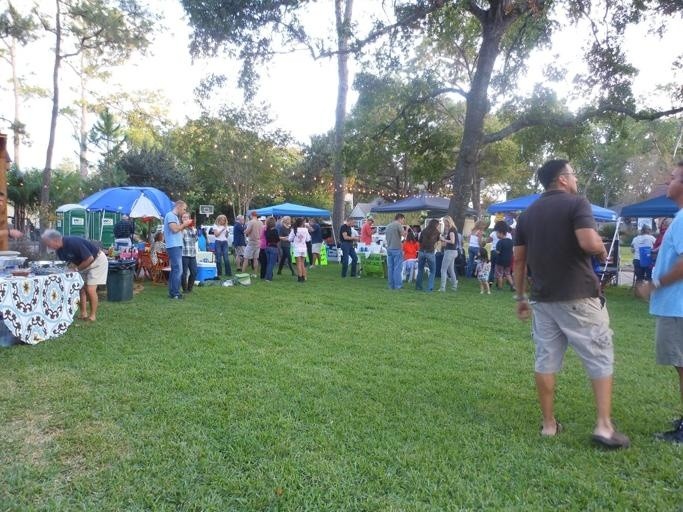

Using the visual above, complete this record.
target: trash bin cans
[106,259,136,302]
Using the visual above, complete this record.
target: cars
[318,224,408,250]
[202,226,235,253]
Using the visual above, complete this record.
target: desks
[0,250,85,345]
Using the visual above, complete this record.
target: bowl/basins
[12,271,27,276]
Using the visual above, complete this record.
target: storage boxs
[194,262,216,283]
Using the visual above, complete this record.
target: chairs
[137,252,169,283]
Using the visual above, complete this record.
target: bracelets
[8,227,11,238]
[75,267,79,274]
[513,294,527,301]
[651,277,661,288]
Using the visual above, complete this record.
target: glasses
[561,168,577,176]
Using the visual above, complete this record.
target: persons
[41,229,109,323]
[648,217,672,272]
[511,156,632,448]
[0,192,23,239]
[630,223,656,281]
[636,160,682,443]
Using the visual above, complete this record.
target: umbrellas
[80,185,173,245]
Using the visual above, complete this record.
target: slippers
[539,423,563,435]
[589,429,633,449]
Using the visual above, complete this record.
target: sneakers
[651,413,683,442]
